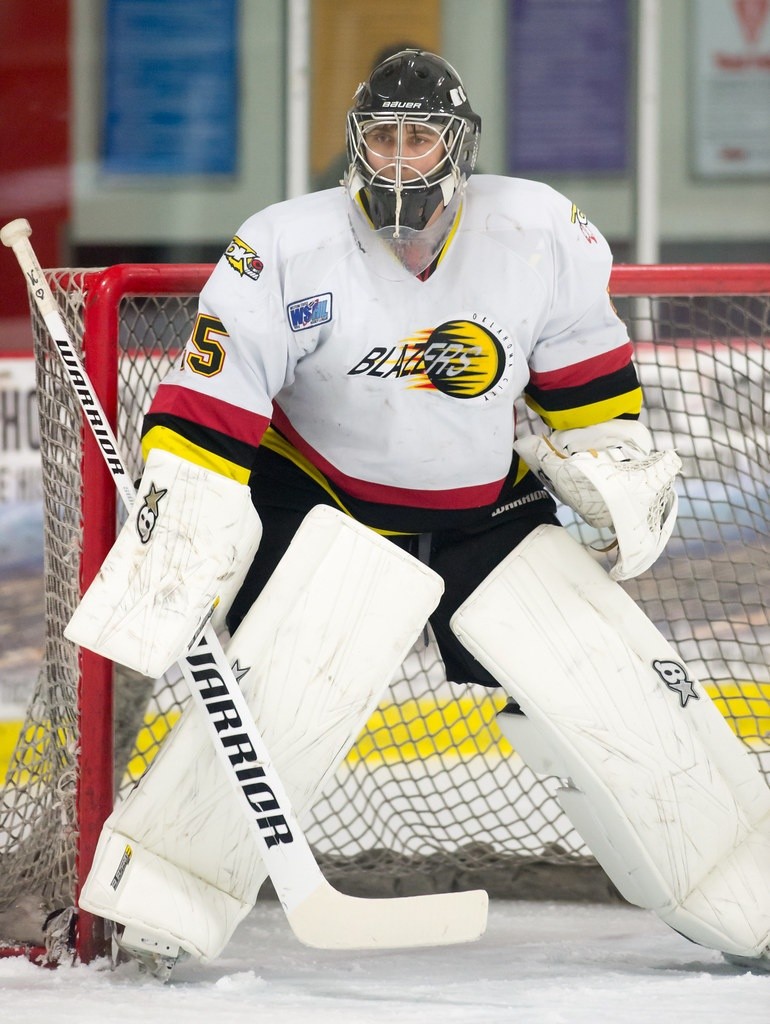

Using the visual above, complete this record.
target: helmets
[343,49,482,239]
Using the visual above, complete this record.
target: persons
[63,48,770,975]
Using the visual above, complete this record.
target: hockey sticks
[1,213,490,953]
